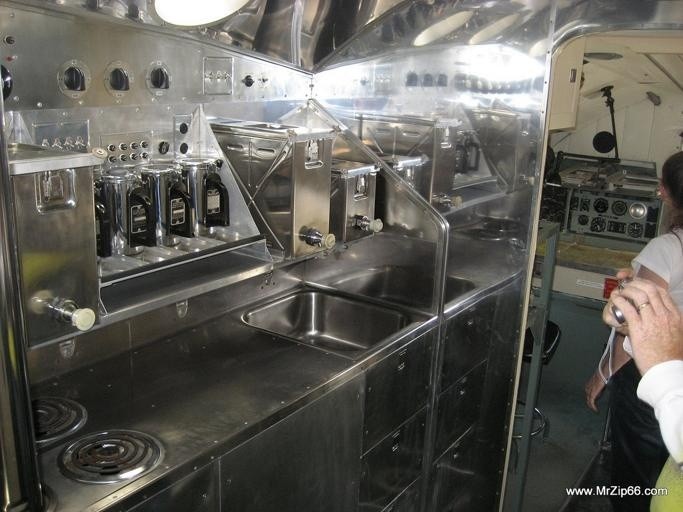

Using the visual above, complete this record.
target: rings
[637,299,649,313]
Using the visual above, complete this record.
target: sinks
[324,262,479,315]
[232,285,437,363]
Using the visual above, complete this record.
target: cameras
[612,278,640,324]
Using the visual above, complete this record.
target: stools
[510,316,561,439]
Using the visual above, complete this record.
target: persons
[598,266,683,512]
[583,146,682,512]
